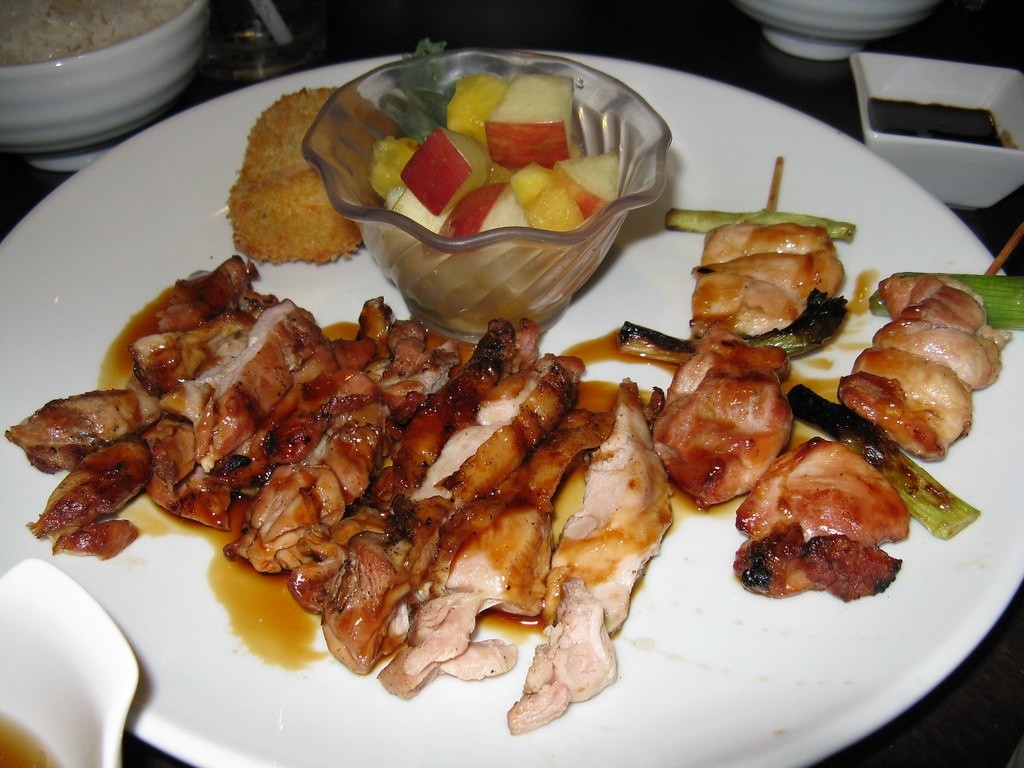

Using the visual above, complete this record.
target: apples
[387,70,620,240]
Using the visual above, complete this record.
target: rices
[0,0,193,66]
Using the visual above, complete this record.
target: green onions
[619,211,1024,536]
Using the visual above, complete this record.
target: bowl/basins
[301,47,673,342]
[0,1,209,184]
[1,557,145,767]
[732,1,945,61]
[851,50,1023,211]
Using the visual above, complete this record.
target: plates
[1,52,1024,768]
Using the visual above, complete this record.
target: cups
[202,1,333,94]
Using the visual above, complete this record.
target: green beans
[379,94,437,143]
